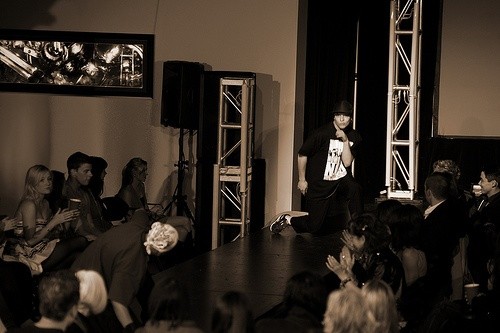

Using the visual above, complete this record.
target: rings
[342,256,345,258]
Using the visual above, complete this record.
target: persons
[270,100,362,234]
[0,151,500,333]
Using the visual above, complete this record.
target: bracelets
[339,277,351,289]
[343,136,347,142]
[44,227,50,232]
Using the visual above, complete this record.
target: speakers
[194,158,266,255]
[160,61,204,130]
[197,71,257,160]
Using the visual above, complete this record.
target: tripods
[161,128,195,223]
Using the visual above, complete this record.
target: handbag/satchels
[0,239,60,281]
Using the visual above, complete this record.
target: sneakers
[270,213,291,234]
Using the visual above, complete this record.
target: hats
[143,222,179,256]
[332,100,353,114]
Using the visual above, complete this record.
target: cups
[473,185,481,192]
[69,199,81,211]
[464,284,480,304]
